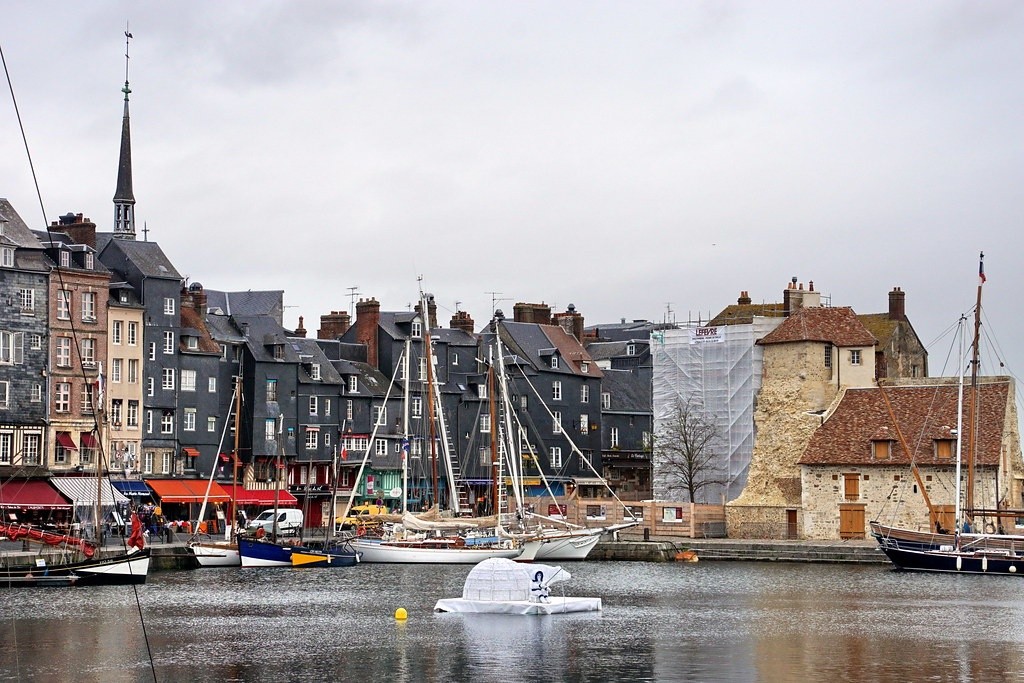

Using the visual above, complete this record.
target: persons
[38,516,56,530]
[532,571,544,582]
[237,511,246,530]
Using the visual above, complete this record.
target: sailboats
[1,272,642,590]
[869,249,1024,578]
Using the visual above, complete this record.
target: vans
[336,505,386,530]
[249,508,304,537]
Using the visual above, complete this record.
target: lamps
[592,423,597,432]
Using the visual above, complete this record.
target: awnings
[144,479,231,503]
[49,477,130,506]
[80,432,98,447]
[56,432,78,452]
[0,479,73,510]
[505,475,608,485]
[109,481,152,496]
[220,484,297,504]
[183,448,284,468]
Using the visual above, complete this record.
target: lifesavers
[358,526,367,536]
[504,540,513,550]
[257,528,265,538]
